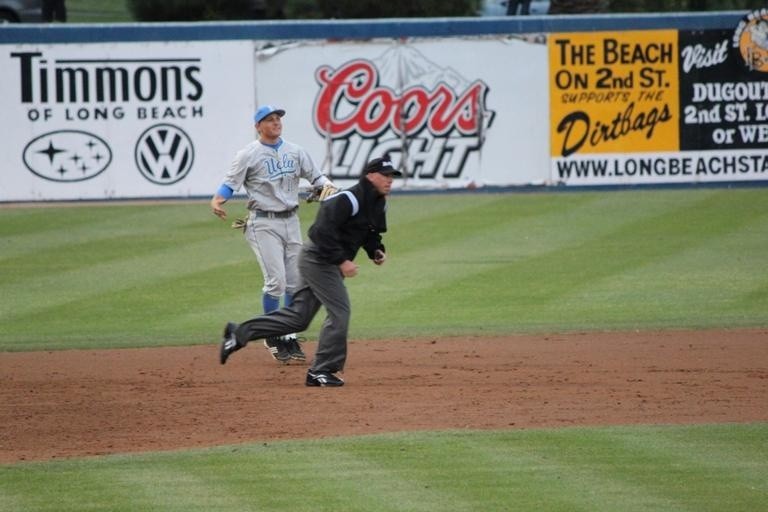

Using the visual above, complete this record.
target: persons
[210,104,342,361]
[220,156,405,389]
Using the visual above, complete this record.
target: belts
[255,210,298,218]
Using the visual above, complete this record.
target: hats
[366,158,401,176]
[254,105,286,122]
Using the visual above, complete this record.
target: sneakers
[220,322,242,364]
[264,337,306,362]
[306,369,343,386]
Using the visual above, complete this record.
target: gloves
[232,219,248,228]
[318,183,339,200]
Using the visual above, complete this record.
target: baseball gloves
[305,186,340,202]
